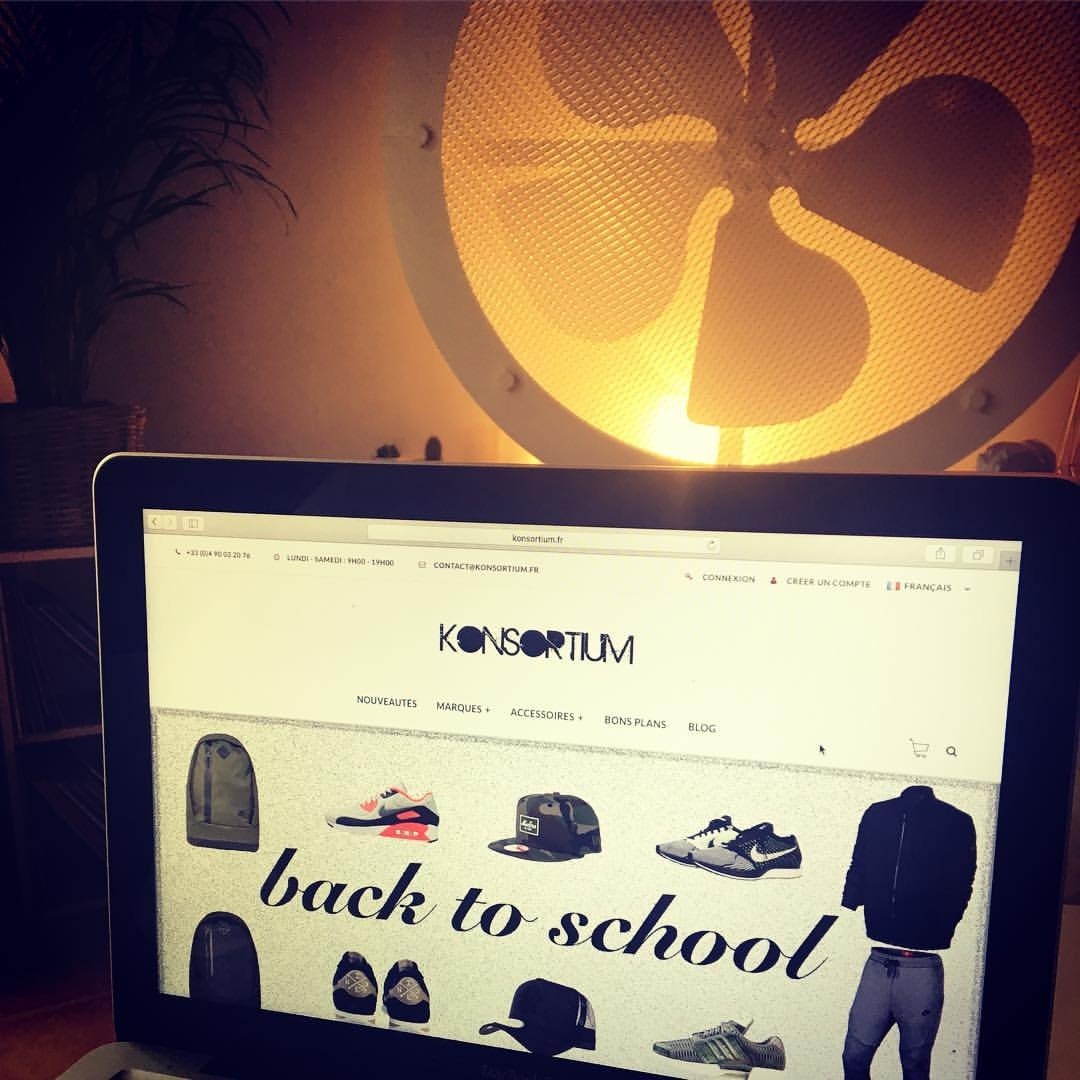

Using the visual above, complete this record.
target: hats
[488,792,601,861]
[479,978,596,1055]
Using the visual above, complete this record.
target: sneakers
[382,960,432,1031]
[655,816,741,865]
[332,951,377,1021]
[688,824,803,879]
[327,781,440,841]
[653,1020,787,1080]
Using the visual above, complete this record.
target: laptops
[51,448,1080,1080]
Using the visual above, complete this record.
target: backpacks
[190,911,260,1011]
[186,733,261,852]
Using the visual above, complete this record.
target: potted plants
[0,0,297,549]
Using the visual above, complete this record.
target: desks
[0,545,110,981]
